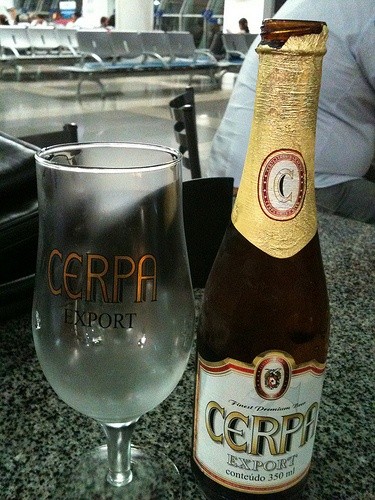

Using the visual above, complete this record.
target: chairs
[168,86,201,179]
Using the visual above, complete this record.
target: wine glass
[31,140,196,500]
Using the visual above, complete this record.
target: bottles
[187,20,332,500]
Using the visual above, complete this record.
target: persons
[237,18,252,36]
[0,8,115,29]
[207,0,375,225]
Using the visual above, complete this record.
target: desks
[0,195,375,500]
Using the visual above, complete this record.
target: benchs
[76,31,219,100]
[218,33,259,85]
[0,28,82,82]
